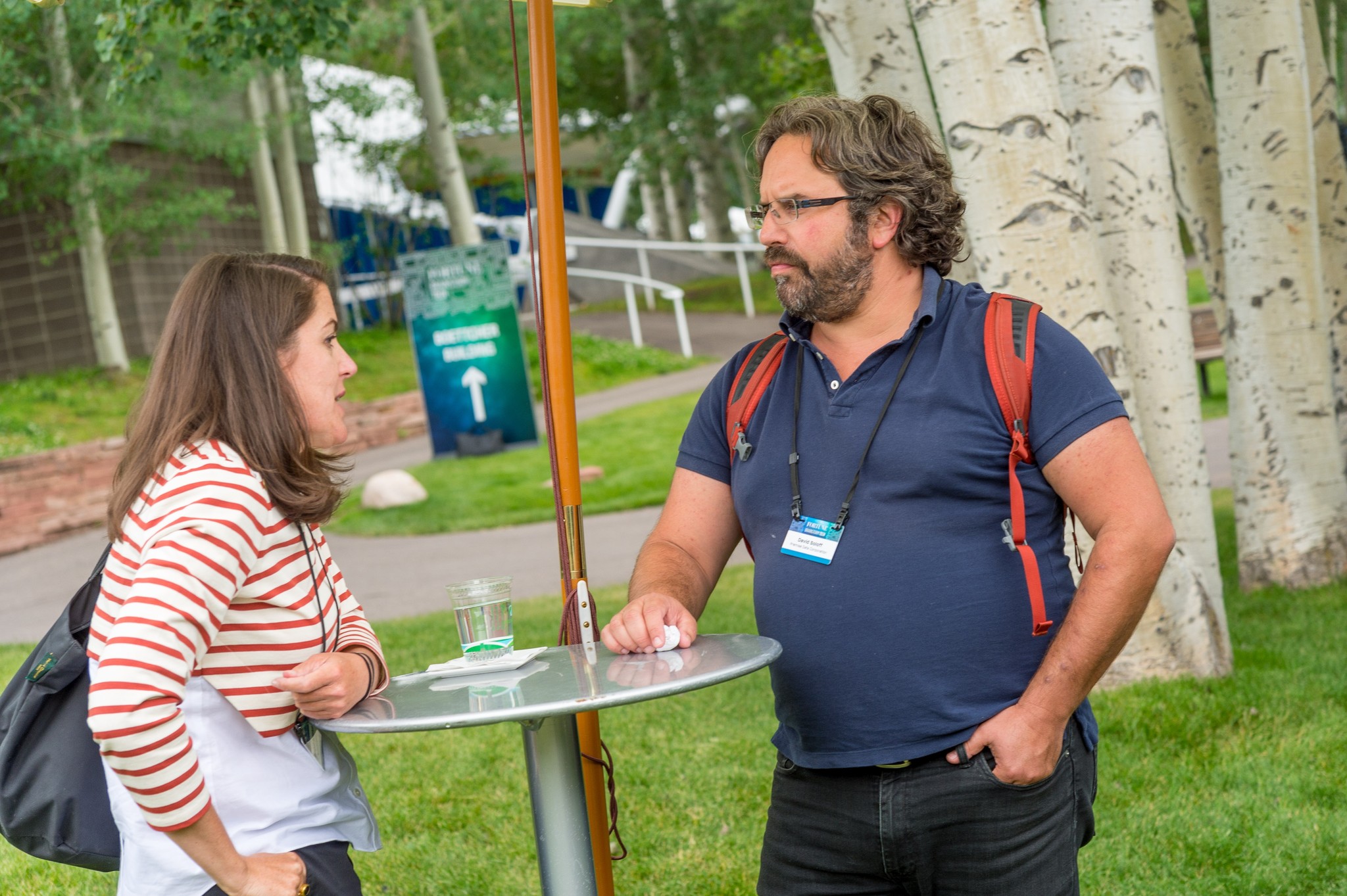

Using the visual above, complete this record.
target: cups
[444,576,514,664]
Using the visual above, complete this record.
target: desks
[308,627,782,895]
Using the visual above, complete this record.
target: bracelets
[351,651,374,703]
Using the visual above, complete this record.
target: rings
[297,884,310,896]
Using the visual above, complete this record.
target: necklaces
[293,521,338,746]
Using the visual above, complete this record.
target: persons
[596,93,1179,896]
[87,251,388,896]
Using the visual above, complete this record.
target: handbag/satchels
[0,504,128,874]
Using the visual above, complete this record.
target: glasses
[745,192,881,233]
